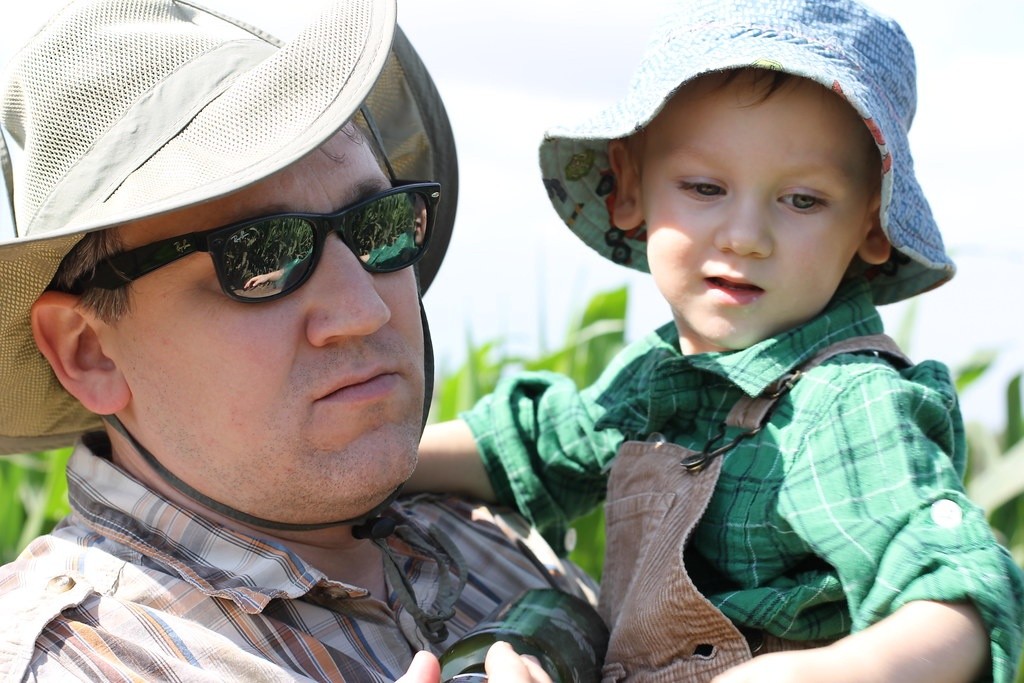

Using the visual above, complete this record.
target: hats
[0,0,459,487]
[539,0,958,307]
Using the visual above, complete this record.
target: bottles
[439,591,610,683]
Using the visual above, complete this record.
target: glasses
[68,182,441,307]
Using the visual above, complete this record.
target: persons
[0,0,604,683]
[398,0,1024,683]
